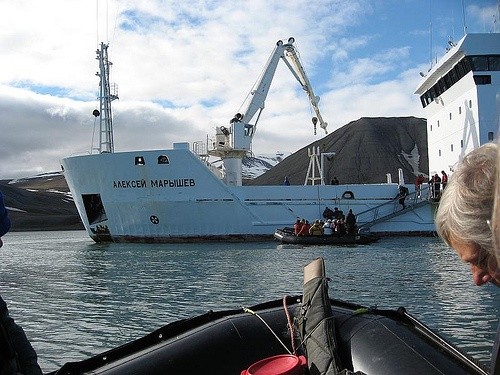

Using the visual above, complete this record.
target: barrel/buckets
[240,354,307,375]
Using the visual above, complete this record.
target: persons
[435,141,500,375]
[346,208,356,236]
[416,173,441,198]
[323,206,334,221]
[284,176,290,185]
[333,207,345,221]
[441,171,448,190]
[294,218,347,236]
[397,185,407,210]
[0,192,43,375]
[331,177,339,185]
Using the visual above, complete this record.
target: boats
[61,0,500,246]
[273,225,379,247]
